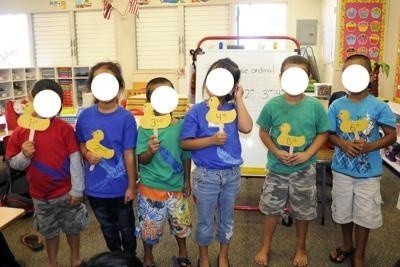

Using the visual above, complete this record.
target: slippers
[173,253,192,267]
[21,233,44,251]
[329,246,354,264]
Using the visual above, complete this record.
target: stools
[317,149,335,225]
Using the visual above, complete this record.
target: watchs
[82,150,89,157]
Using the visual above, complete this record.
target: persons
[326,54,397,267]
[135,78,192,267]
[73,250,144,267]
[75,62,138,256]
[256,56,333,267]
[5,79,90,267]
[179,58,253,267]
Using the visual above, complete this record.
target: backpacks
[6,100,31,130]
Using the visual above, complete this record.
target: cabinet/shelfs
[1,66,97,116]
[377,96,400,175]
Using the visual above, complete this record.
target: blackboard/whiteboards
[190,49,302,177]
